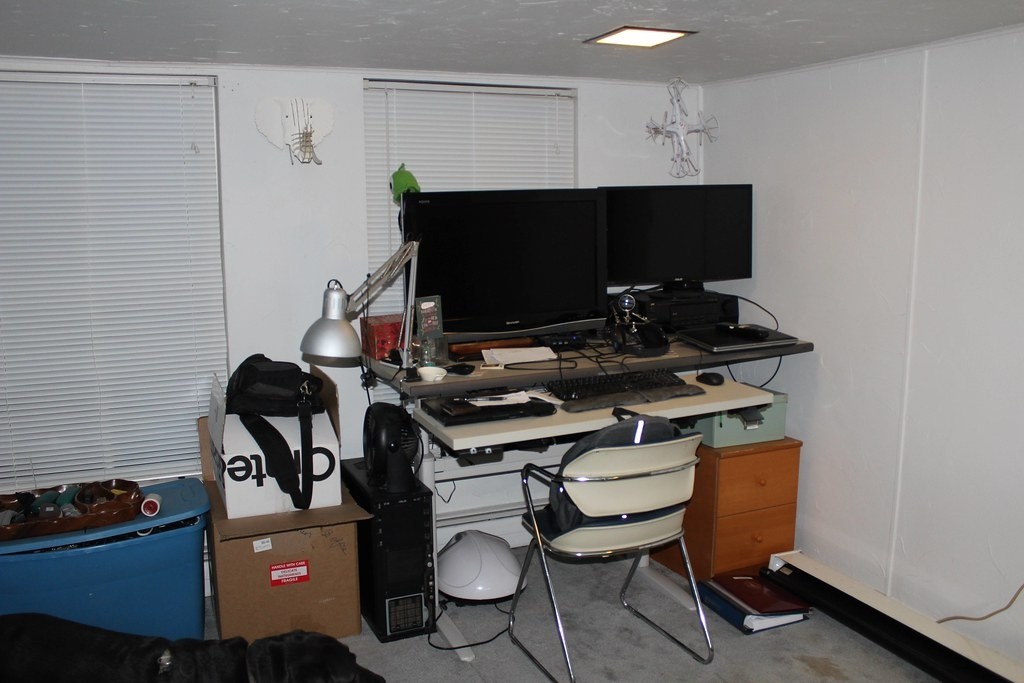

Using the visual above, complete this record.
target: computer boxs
[340,457,436,643]
[607,289,739,334]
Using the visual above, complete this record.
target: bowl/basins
[418,367,447,381]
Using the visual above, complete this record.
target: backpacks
[548,408,686,539]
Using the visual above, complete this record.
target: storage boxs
[0,478,211,641]
[207,357,342,521]
[196,415,375,646]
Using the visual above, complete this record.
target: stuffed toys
[390,163,420,206]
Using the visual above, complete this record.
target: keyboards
[547,367,686,401]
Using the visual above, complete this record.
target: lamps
[300,240,421,369]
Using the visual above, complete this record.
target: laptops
[676,324,799,353]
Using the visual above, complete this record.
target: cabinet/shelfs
[672,384,788,449]
[649,436,803,582]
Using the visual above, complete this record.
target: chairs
[509,416,716,683]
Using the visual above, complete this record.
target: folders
[693,569,814,634]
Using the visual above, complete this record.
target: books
[699,572,811,635]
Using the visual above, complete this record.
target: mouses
[696,372,724,385]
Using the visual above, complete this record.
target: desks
[362,325,814,662]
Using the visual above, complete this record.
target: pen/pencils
[453,396,506,401]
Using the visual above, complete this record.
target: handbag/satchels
[225,354,326,515]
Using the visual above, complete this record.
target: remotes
[716,322,769,340]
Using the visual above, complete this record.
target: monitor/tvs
[401,189,608,362]
[596,184,753,299]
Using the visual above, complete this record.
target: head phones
[611,322,669,358]
